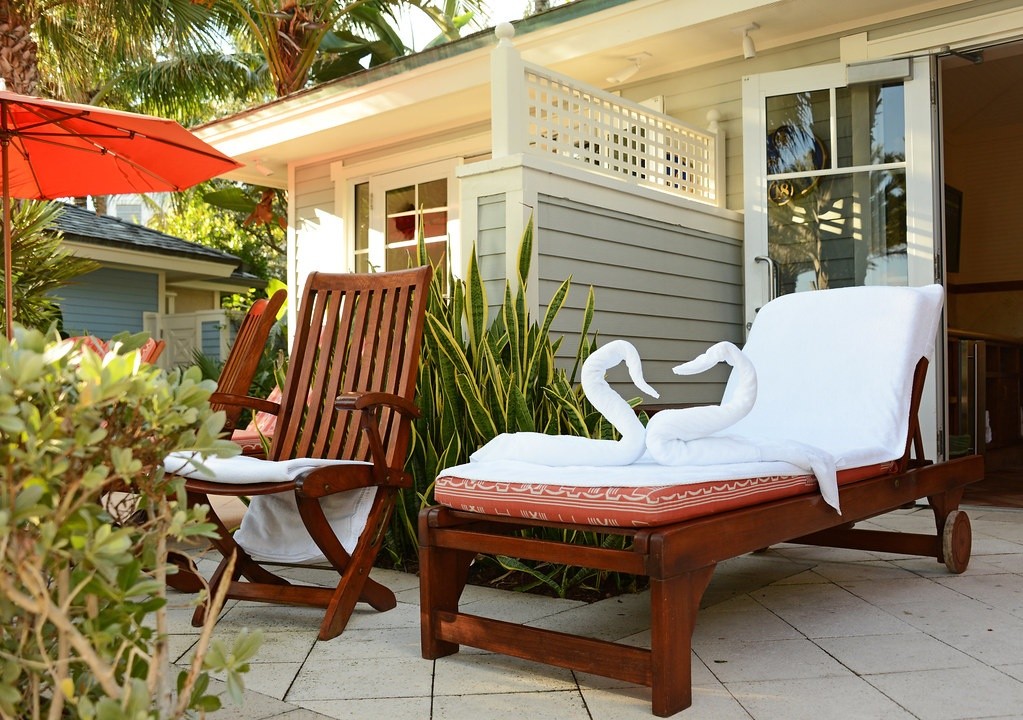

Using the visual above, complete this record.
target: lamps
[742,22,760,60]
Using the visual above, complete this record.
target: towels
[469,340,659,466]
[432,280,945,486]
[157,451,378,563]
[646,341,841,515]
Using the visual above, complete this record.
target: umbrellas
[0,90,246,343]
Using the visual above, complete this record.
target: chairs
[419,282,974,717]
[105,287,288,593]
[156,262,434,643]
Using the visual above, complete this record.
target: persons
[38,305,70,341]
